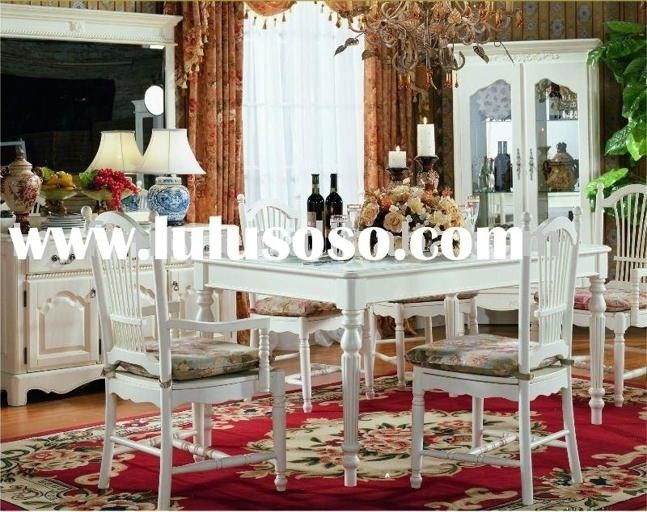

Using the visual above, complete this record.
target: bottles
[477,157,490,194]
[493,142,506,191]
[324,173,344,253]
[306,174,324,253]
[502,141,513,191]
[487,158,495,194]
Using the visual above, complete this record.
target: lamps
[333,0,525,101]
[141,129,206,226]
[85,130,144,213]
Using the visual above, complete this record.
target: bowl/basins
[0,217,16,234]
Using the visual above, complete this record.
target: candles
[417,116,435,157]
[388,145,407,169]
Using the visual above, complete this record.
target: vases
[0,144,44,223]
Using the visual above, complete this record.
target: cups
[346,203,362,243]
[328,213,350,263]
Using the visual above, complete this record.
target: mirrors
[0,3,184,229]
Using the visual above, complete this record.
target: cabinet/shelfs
[447,39,603,330]
[0,223,237,406]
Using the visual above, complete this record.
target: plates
[479,83,511,120]
[42,214,86,230]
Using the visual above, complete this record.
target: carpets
[0,362,647,511]
